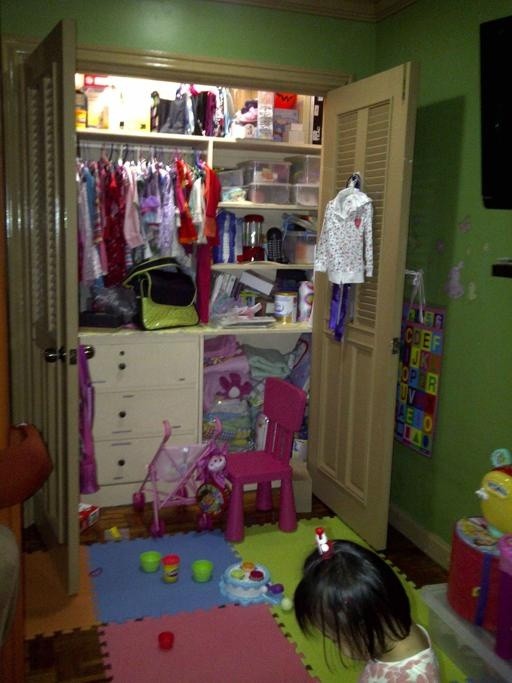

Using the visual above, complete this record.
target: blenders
[241,215,266,262]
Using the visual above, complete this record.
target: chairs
[203,378,307,543]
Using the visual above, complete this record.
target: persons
[292,525,442,683]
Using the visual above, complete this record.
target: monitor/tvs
[479,12,512,209]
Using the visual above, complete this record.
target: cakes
[219,561,273,605]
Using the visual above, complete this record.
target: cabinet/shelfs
[73,72,323,508]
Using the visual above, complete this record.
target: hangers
[76,141,214,185]
[326,173,373,208]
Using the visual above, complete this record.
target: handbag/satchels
[137,269,200,331]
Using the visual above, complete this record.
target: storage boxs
[216,153,321,207]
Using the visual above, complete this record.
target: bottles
[160,555,180,584]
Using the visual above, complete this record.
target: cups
[191,559,214,582]
[157,630,175,651]
[140,550,162,573]
